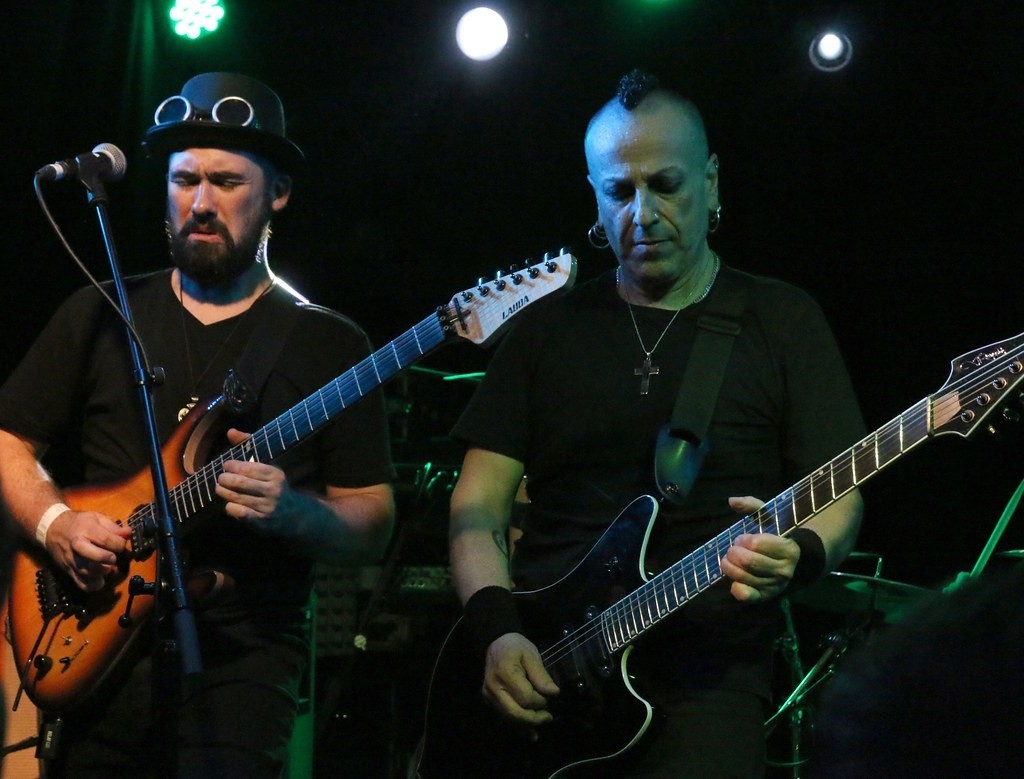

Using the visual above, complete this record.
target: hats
[146,72,309,180]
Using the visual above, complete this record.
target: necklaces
[616,249,718,304]
[178,269,272,420]
[622,249,710,395]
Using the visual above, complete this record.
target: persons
[448,67,866,779]
[1,73,397,778]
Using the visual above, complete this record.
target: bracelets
[34,502,72,550]
[784,527,825,595]
[464,586,522,655]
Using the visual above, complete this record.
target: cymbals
[790,556,934,624]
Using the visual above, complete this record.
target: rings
[89,560,94,568]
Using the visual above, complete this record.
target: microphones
[37,142,127,182]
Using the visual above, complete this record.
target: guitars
[421,333,1024,779]
[5,244,581,729]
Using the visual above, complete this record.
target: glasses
[153,95,260,127]
